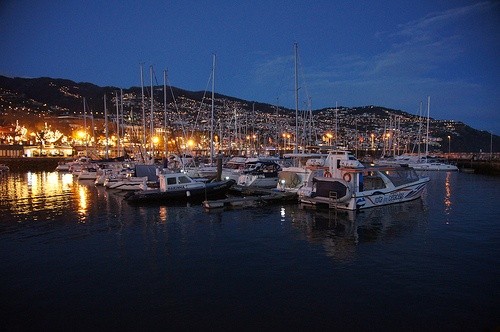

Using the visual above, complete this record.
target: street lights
[371,133,375,148]
[386,133,390,149]
[448,136,451,154]
[283,134,286,148]
[247,136,250,144]
[327,133,333,145]
[253,135,256,146]
[287,135,290,148]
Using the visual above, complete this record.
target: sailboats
[56,38,459,212]
